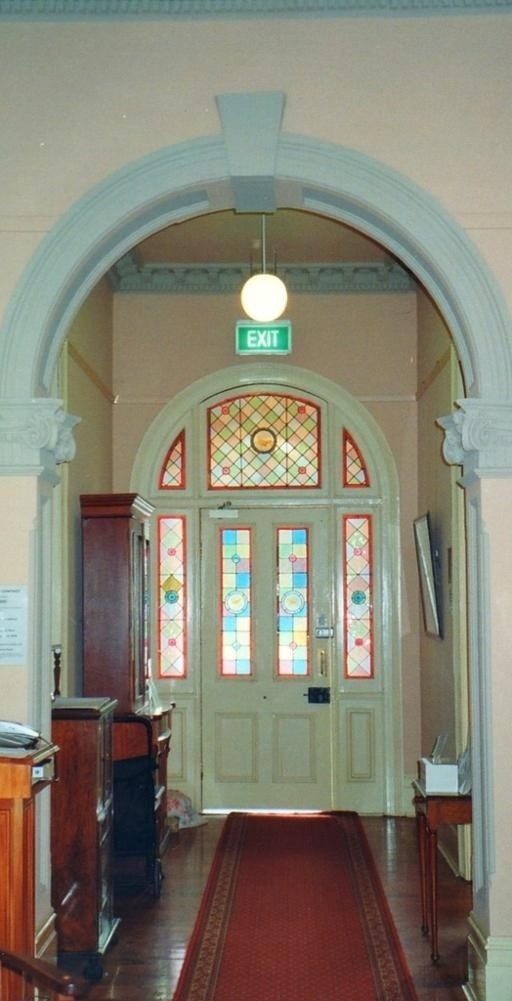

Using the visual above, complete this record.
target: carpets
[175,810,423,999]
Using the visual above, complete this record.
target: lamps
[239,212,290,324]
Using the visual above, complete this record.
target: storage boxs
[417,756,459,793]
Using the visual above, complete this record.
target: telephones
[0,720,41,750]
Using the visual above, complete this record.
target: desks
[403,779,472,962]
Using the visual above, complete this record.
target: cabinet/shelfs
[108,699,174,863]
[79,492,159,717]
[0,745,61,1001]
[52,697,125,959]
